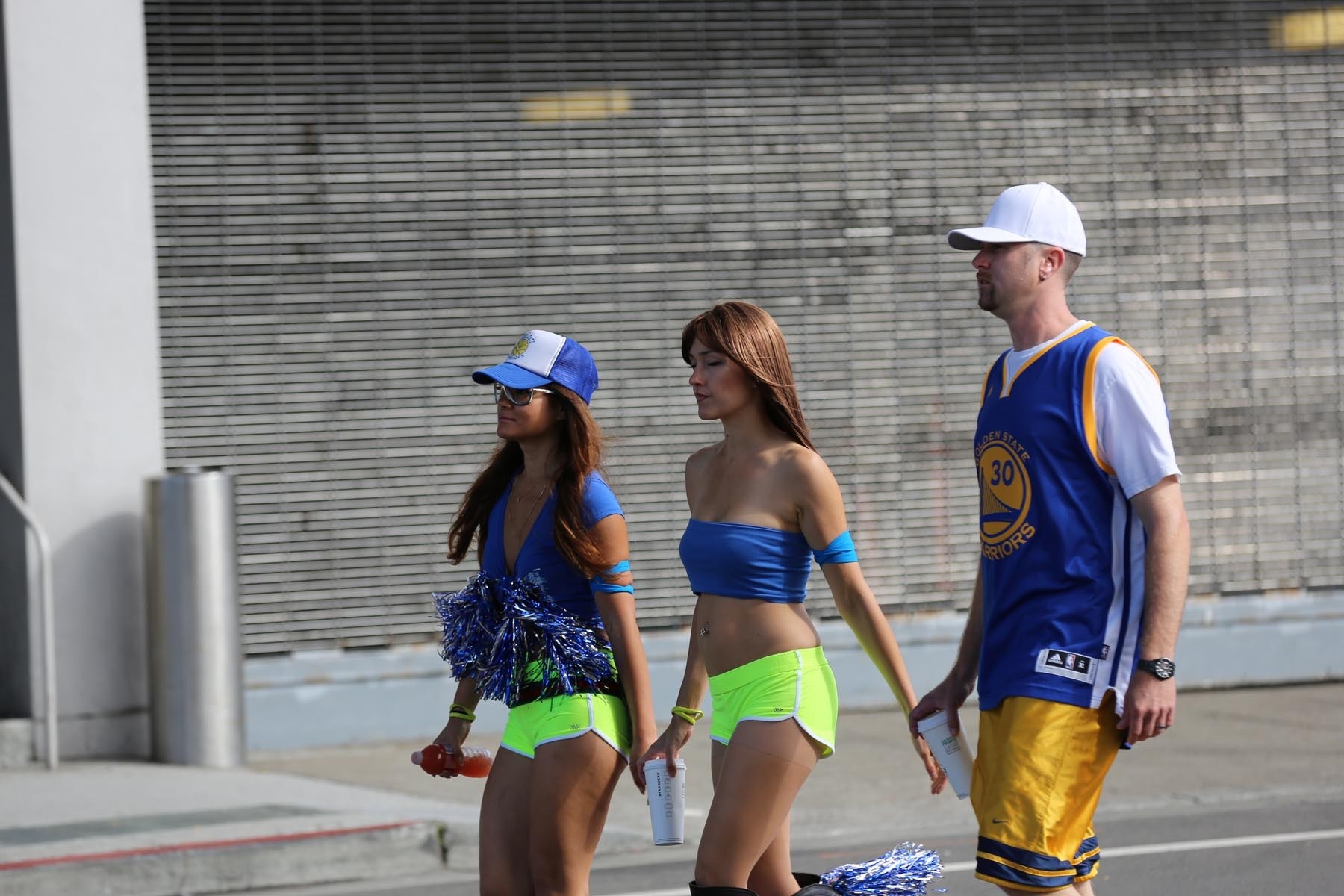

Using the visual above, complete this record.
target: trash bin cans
[147,466,243,767]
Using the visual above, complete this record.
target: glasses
[492,380,556,406]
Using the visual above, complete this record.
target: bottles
[411,744,495,779]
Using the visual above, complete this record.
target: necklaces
[507,486,546,541]
[513,481,538,502]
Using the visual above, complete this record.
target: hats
[948,182,1086,256]
[472,330,599,405]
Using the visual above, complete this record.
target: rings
[1155,723,1166,729]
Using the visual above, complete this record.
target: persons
[636,301,947,896]
[909,181,1191,896]
[420,329,661,896]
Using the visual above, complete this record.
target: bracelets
[449,703,476,723]
[674,711,696,726]
[672,706,704,720]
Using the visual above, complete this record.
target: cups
[913,709,979,800]
[643,758,686,846]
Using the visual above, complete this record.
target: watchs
[1137,656,1176,680]
